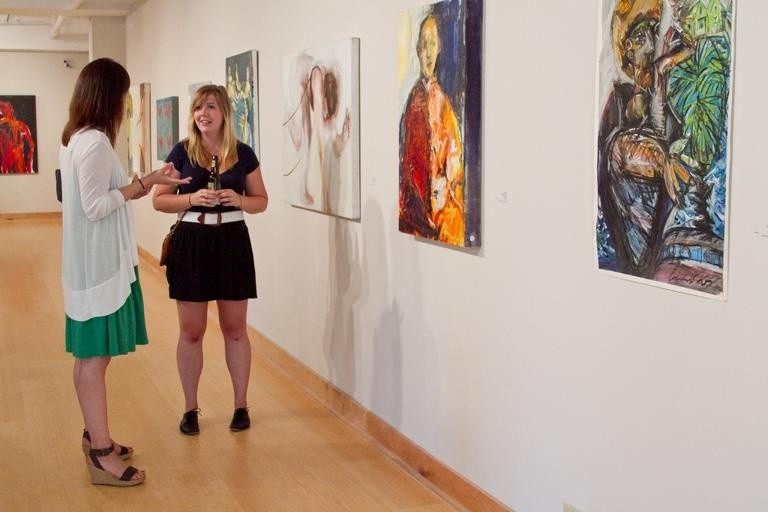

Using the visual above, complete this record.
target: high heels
[81,430,146,486]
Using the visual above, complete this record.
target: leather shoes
[179,412,200,436]
[177,210,245,224]
[229,408,249,431]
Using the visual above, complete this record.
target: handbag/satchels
[159,223,177,266]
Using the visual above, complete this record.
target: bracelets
[138,179,146,193]
[188,192,193,207]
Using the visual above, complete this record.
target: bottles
[208,155,221,206]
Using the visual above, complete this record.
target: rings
[200,194,208,198]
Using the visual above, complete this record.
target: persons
[152,84,268,435]
[395,12,465,245]
[58,58,193,486]
